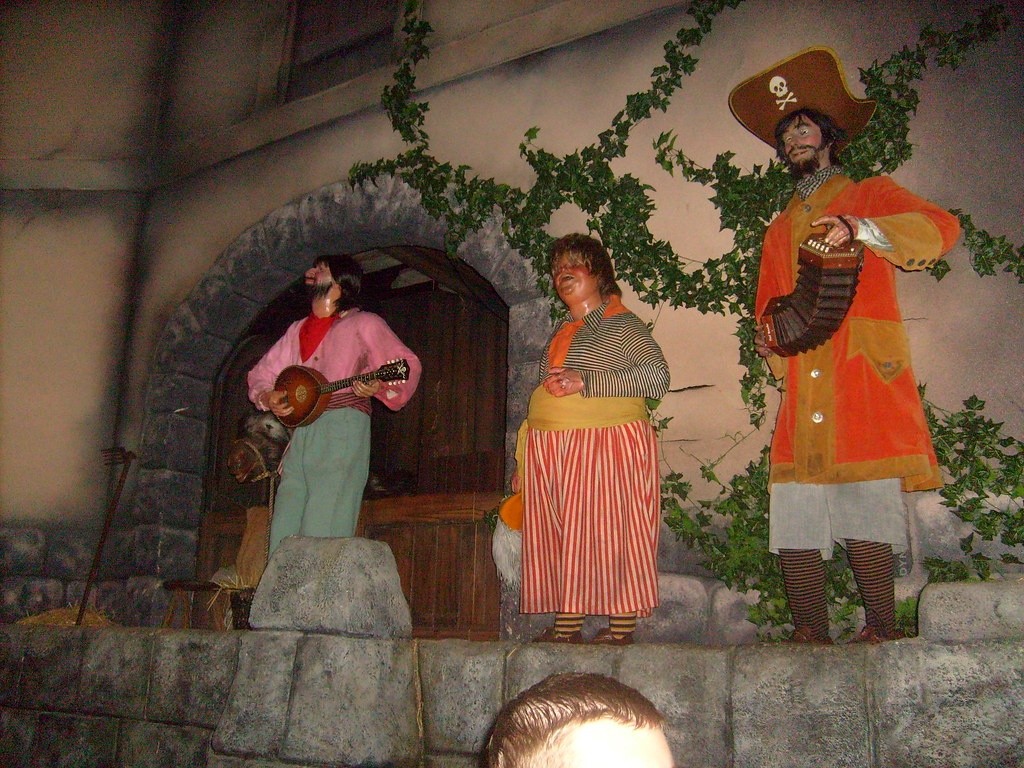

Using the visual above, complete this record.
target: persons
[510,233,670,645]
[485,672,675,768]
[729,50,961,646]
[247,253,422,560]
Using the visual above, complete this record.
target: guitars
[269,353,411,428]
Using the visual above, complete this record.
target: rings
[557,373,565,389]
